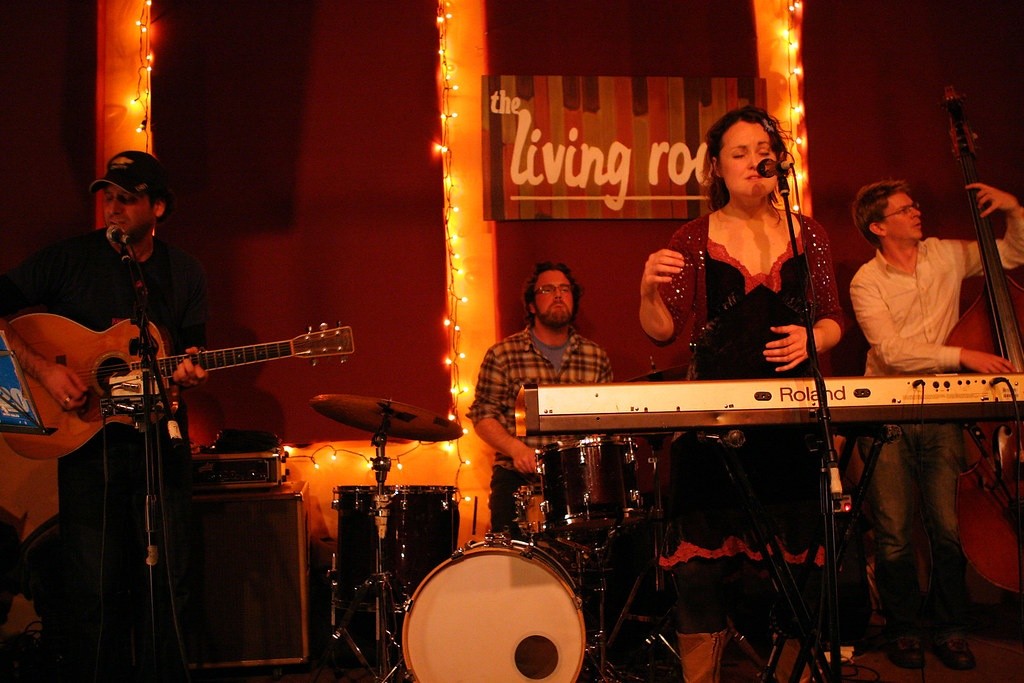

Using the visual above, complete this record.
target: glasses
[882,202,919,219]
[535,284,573,296]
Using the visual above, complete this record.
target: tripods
[309,420,396,683]
[606,433,765,683]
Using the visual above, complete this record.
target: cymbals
[309,391,464,443]
[623,361,690,383]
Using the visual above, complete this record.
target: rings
[64,396,71,405]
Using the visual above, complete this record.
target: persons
[850,179,1023,668]
[469,254,615,582]
[0,151,211,683]
[631,108,846,682]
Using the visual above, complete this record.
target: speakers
[180,484,309,672]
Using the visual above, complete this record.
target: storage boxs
[194,484,309,669]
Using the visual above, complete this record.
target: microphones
[755,158,795,178]
[106,225,131,245]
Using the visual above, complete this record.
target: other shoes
[929,630,977,670]
[888,636,925,669]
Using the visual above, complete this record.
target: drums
[335,482,461,608]
[514,482,550,531]
[399,533,593,683]
[536,439,648,531]
[511,367,1024,683]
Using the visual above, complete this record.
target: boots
[676,628,728,683]
[772,632,815,682]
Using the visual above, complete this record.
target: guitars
[1,312,356,460]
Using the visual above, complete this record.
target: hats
[88,150,168,200]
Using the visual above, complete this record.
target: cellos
[942,86,1024,594]
